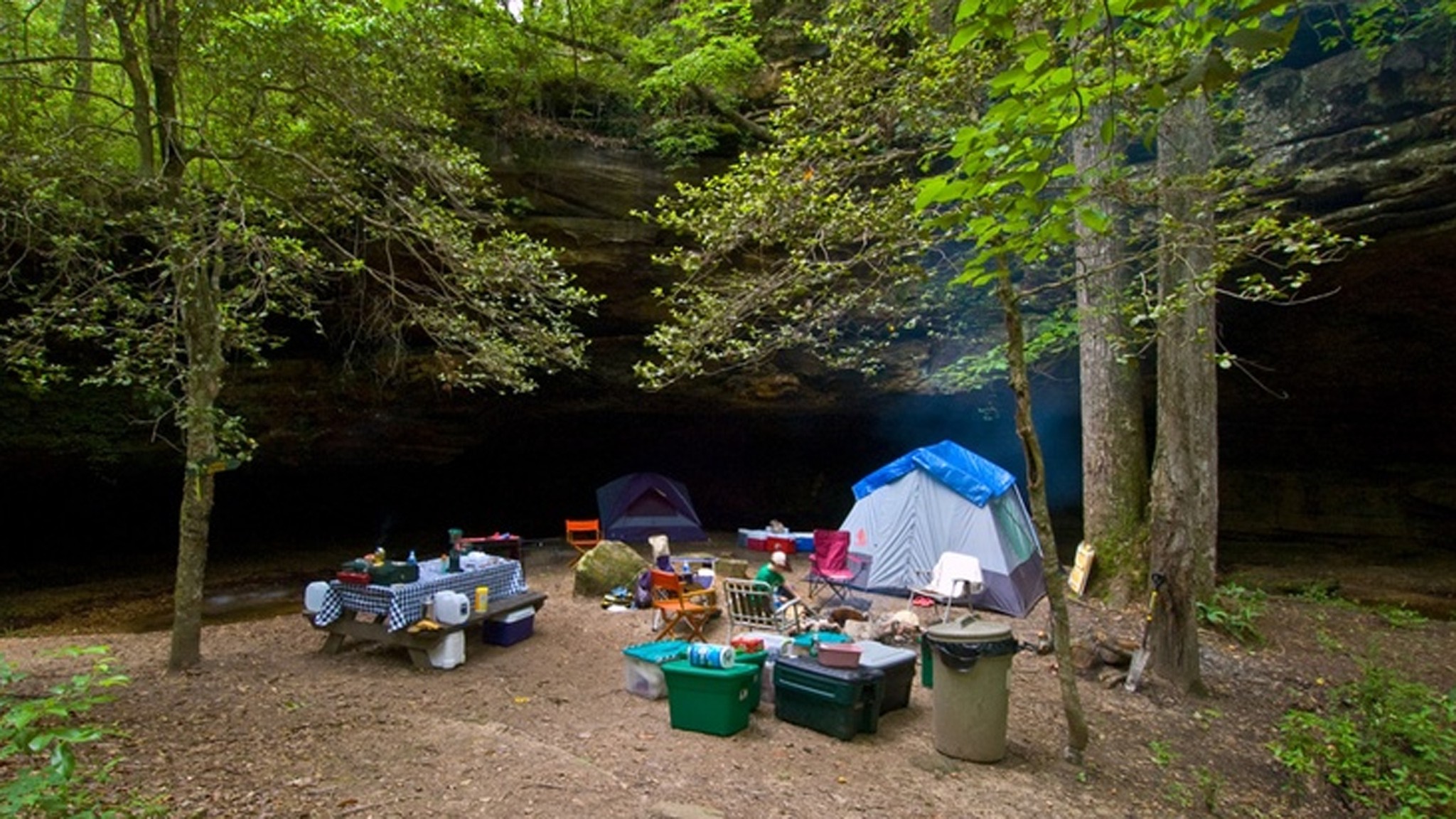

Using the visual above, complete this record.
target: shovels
[1123,573,1166,692]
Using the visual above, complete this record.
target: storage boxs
[737,528,815,553]
[483,605,537,646]
[623,630,916,739]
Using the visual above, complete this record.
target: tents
[803,440,1047,620]
[595,470,709,544]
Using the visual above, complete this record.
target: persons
[748,550,798,620]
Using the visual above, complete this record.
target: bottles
[408,551,416,566]
[438,554,447,574]
[683,561,690,574]
[449,547,459,571]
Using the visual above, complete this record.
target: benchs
[408,591,548,670]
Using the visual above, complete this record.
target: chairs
[724,577,802,645]
[906,551,985,623]
[809,529,866,601]
[653,570,717,641]
[566,520,600,570]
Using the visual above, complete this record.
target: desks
[456,536,523,570]
[320,549,524,669]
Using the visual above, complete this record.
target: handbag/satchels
[809,528,855,580]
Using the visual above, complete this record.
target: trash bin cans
[927,620,1019,761]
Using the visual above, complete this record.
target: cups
[686,642,736,669]
[475,587,489,614]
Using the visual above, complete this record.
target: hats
[770,551,793,572]
